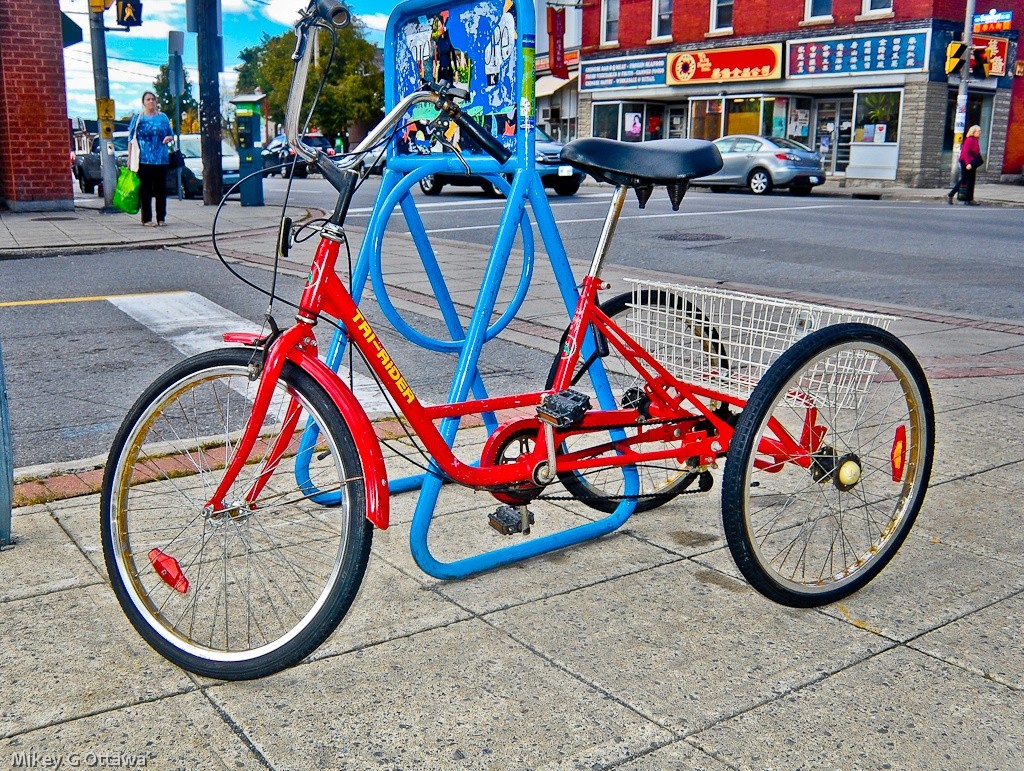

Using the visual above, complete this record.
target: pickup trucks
[72,131,129,197]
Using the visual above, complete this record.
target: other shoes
[967,200,980,206]
[945,193,953,205]
[156,220,167,226]
[144,221,156,227]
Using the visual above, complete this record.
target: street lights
[253,87,268,144]
[182,112,193,133]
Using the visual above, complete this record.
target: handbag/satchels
[166,140,185,170]
[130,114,142,172]
[970,155,984,171]
[957,182,968,201]
[113,166,141,215]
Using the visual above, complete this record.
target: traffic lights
[972,49,991,78]
[116,0,143,26]
[945,43,968,74]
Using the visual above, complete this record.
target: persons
[128,92,175,226]
[946,124,982,205]
[632,115,641,133]
[434,16,459,101]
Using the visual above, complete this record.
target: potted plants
[865,93,896,142]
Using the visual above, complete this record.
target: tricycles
[99,0,936,680]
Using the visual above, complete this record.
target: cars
[165,134,241,200]
[261,133,335,179]
[420,125,586,198]
[689,134,827,196]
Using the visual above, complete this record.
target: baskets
[620,276,903,409]
